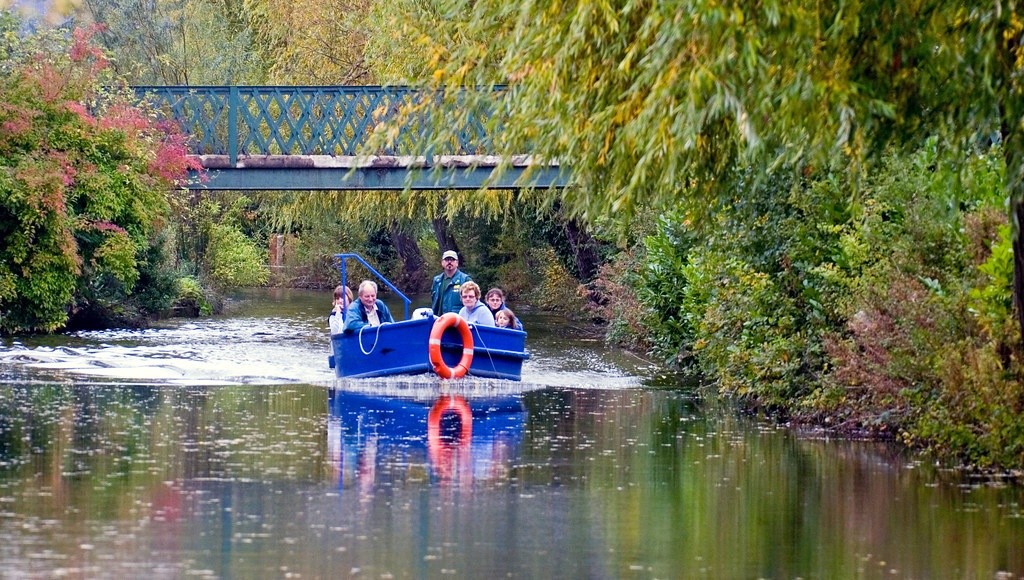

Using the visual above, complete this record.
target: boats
[326,251,531,382]
[326,387,528,497]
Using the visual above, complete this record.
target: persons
[459,281,495,326]
[328,285,354,334]
[485,288,523,331]
[496,310,515,329]
[430,249,473,317]
[342,280,393,333]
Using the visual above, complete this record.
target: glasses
[461,295,474,298]
[443,257,456,261]
[489,298,499,302]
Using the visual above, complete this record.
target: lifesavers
[427,311,475,380]
[426,394,473,463]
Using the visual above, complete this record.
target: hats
[442,250,458,260]
